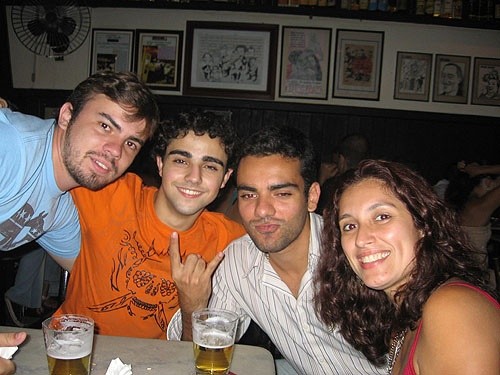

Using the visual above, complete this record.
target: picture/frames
[279,26,332,100]
[393,51,433,102]
[134,29,184,91]
[90,27,135,77]
[183,20,279,101]
[432,54,471,104]
[332,28,385,101]
[471,57,500,107]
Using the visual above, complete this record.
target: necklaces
[386,330,408,374]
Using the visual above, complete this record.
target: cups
[42,314,95,375]
[191,308,239,375]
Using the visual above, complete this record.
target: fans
[11,0,91,82]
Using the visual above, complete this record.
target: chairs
[5,247,47,327]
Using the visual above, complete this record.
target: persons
[49,108,247,340]
[166,126,500,375]
[0,70,159,375]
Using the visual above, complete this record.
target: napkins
[0,346,18,359]
[106,358,133,375]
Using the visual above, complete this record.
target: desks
[0,325,276,375]
